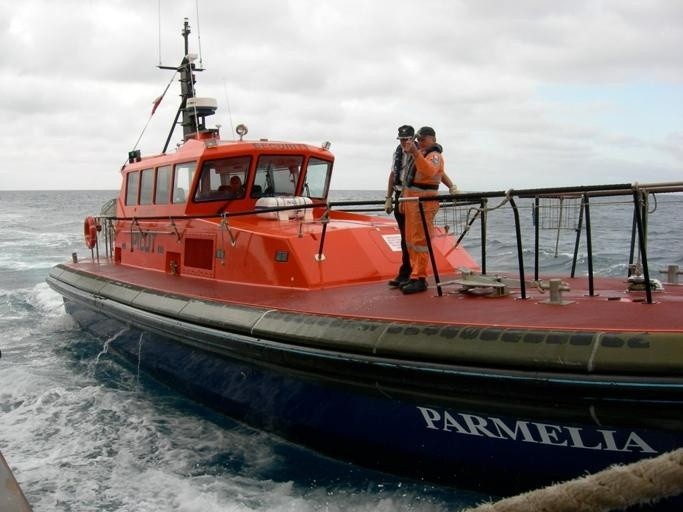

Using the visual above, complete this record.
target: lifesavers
[84,216,96,250]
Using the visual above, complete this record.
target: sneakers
[387,275,427,294]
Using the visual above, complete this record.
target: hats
[397,125,414,139]
[414,126,435,140]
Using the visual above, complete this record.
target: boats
[45,14,683,495]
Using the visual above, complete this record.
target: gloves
[449,185,466,195]
[384,197,393,215]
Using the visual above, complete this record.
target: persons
[383,124,459,287]
[399,126,444,295]
[223,175,246,198]
